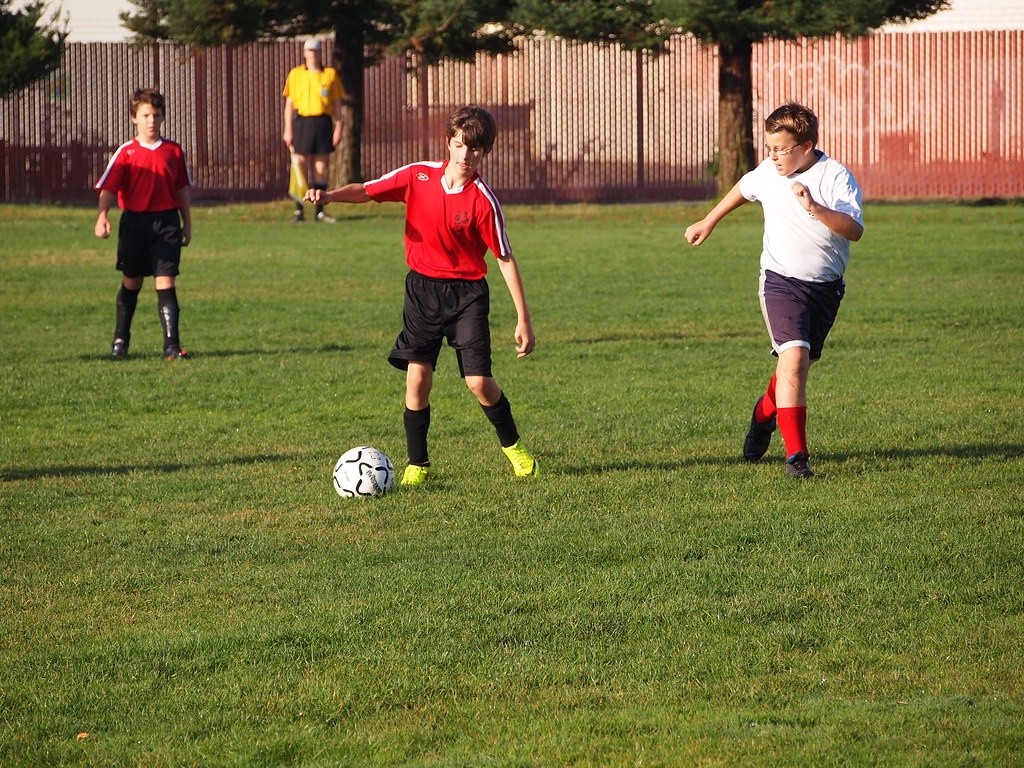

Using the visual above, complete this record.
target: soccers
[332,446,395,498]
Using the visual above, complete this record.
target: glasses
[765,140,803,155]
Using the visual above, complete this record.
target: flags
[288,154,309,205]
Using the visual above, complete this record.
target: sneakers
[501,437,541,480]
[398,464,430,490]
[743,398,778,464]
[784,449,813,479]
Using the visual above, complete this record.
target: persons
[282,39,345,224]
[95,89,194,360]
[684,104,864,479]
[302,104,540,486]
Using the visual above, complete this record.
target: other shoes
[291,210,304,223]
[111,338,128,360]
[162,344,190,361]
[314,213,336,224]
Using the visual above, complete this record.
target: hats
[303,39,321,51]
